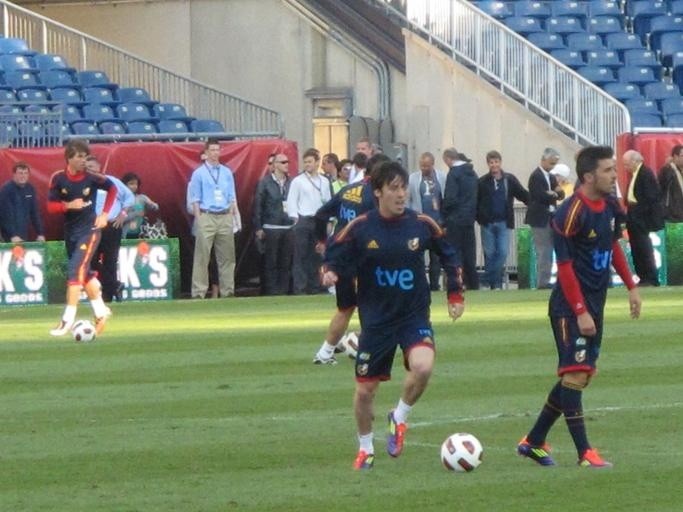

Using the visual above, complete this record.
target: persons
[0,161,45,244]
[183,135,683,299]
[45,140,117,336]
[119,171,160,240]
[82,156,134,306]
[517,144,645,470]
[313,155,393,363]
[320,160,465,471]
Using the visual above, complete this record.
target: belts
[200,209,230,215]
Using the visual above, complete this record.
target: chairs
[467,1,683,127]
[0,33,233,144]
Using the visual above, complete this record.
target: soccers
[73,319,95,341]
[440,433,483,471]
[344,333,359,358]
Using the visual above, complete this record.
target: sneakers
[94,306,112,333]
[577,447,613,468]
[353,450,374,469]
[517,435,555,466]
[387,410,407,457]
[312,346,335,364]
[49,320,73,336]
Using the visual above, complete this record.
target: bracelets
[120,210,129,219]
